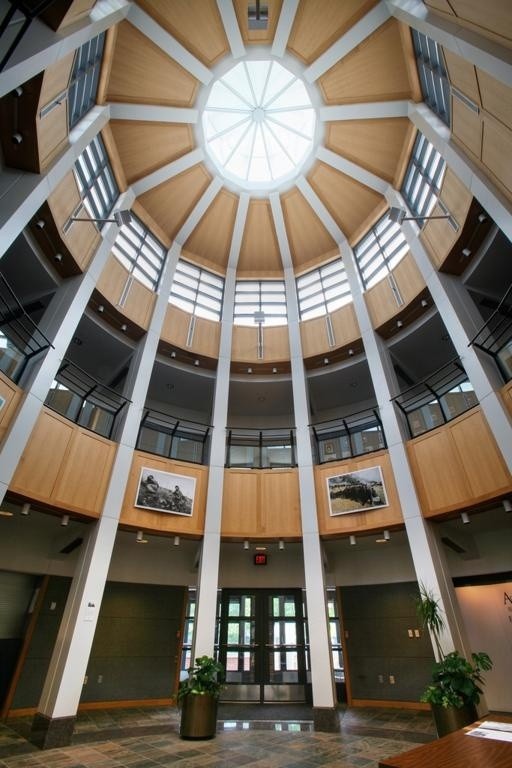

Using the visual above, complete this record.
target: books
[464,720,512,743]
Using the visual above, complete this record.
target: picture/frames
[131,465,198,518]
[326,465,389,516]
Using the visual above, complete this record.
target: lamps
[137,530,143,541]
[461,212,487,257]
[248,366,252,373]
[422,300,427,306]
[8,83,66,269]
[171,351,175,358]
[20,503,31,516]
[121,324,127,331]
[173,535,180,546]
[504,501,512,511]
[461,512,470,524]
[243,539,249,549]
[350,535,355,544]
[324,357,329,363]
[349,348,354,354]
[272,366,277,373]
[397,320,403,328]
[246,0,270,33]
[60,513,71,526]
[195,359,199,365]
[387,200,459,232]
[384,530,389,540]
[279,540,285,550]
[62,199,132,236]
[98,305,103,312]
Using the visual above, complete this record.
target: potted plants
[415,585,493,738]
[177,656,222,739]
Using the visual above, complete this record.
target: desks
[377,717,510,767]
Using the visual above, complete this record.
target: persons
[331,484,373,506]
[147,475,186,512]
